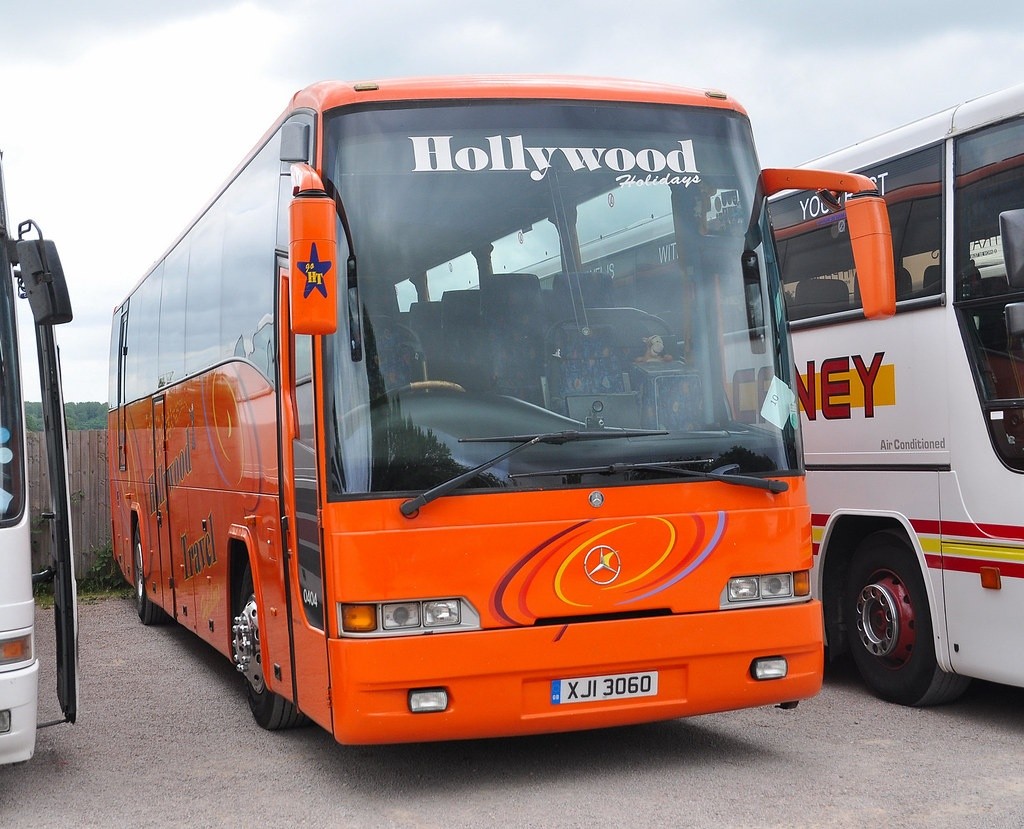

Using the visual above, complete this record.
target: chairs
[791,258,1023,398]
[347,268,646,412]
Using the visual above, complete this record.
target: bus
[503,83,1024,714]
[112,84,901,752]
[1,148,84,768]
